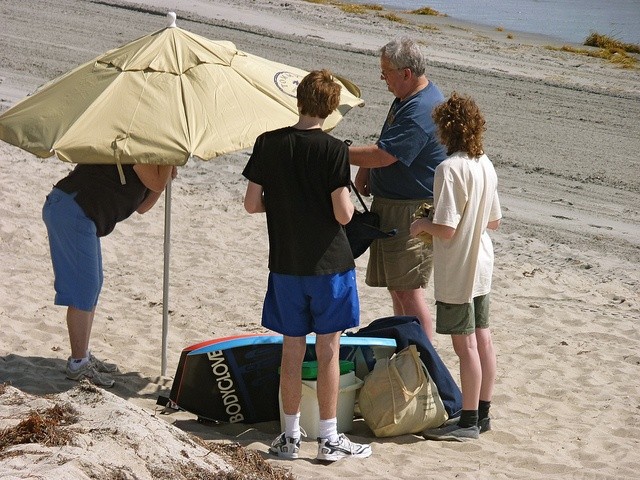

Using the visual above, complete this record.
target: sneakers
[317,433,372,461]
[66,361,115,388]
[271,426,307,459]
[65,352,117,373]
[441,416,490,434]
[422,423,482,442]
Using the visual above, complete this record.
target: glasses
[381,67,413,80]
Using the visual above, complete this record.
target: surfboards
[175,337,399,424]
[168,333,283,402]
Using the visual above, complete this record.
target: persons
[41,160,178,390]
[348,35,449,341]
[240,67,373,461]
[408,92,503,443]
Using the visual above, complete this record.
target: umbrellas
[0,9,369,377]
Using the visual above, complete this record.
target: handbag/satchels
[345,316,463,419]
[359,345,449,438]
[341,181,398,259]
